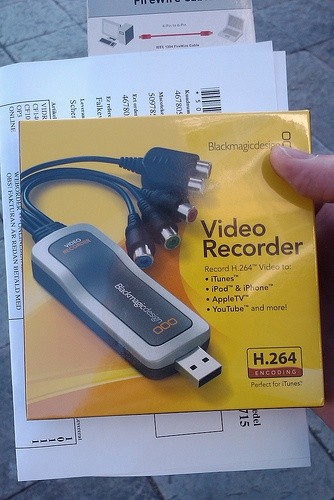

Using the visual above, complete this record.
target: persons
[269,144,334,432]
